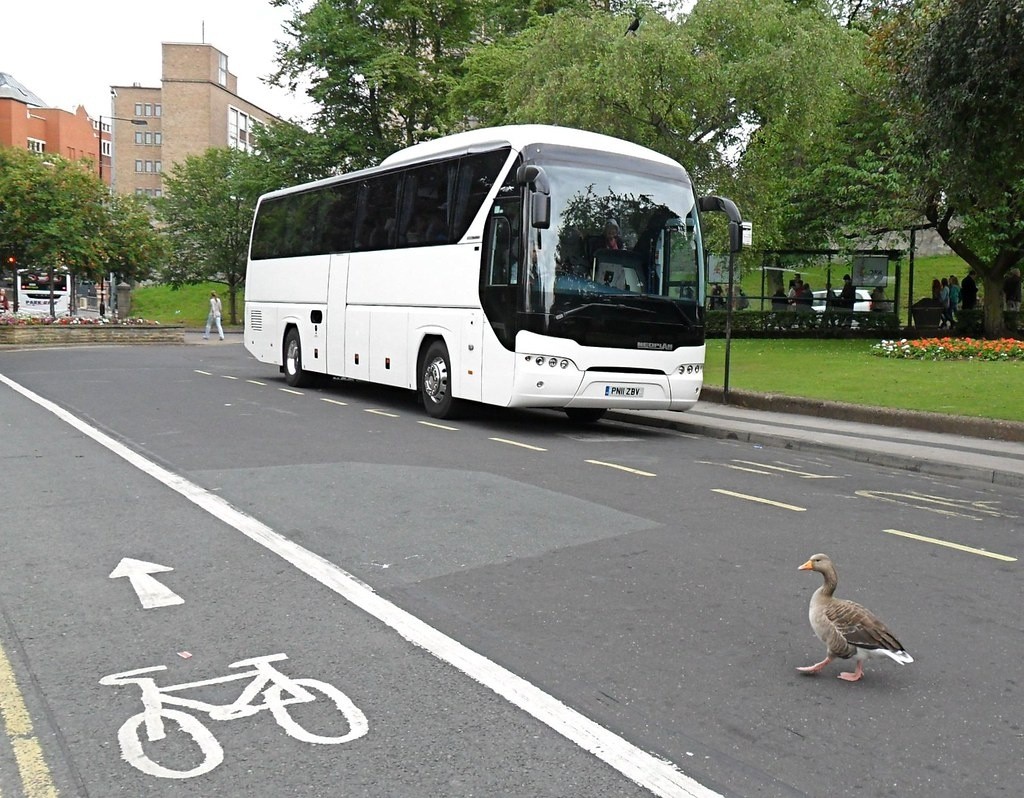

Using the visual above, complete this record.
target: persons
[510,242,562,287]
[592,219,628,251]
[1003,268,1021,311]
[685,286,696,301]
[933,270,979,328]
[872,287,885,324]
[0,288,9,312]
[709,283,745,310]
[406,216,428,242]
[825,275,856,327]
[203,290,224,340]
[772,274,813,329]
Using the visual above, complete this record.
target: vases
[0,325,185,350]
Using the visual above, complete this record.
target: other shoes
[949,321,956,329]
[219,339,224,341]
[203,336,208,340]
[938,324,946,329]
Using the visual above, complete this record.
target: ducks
[791,553,915,682]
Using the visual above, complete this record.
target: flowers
[0,310,161,326]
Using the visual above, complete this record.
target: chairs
[369,226,386,247]
[426,216,448,241]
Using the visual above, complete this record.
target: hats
[602,219,619,232]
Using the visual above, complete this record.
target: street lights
[97,116,148,315]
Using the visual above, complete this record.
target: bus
[14,266,71,318]
[243,123,752,425]
